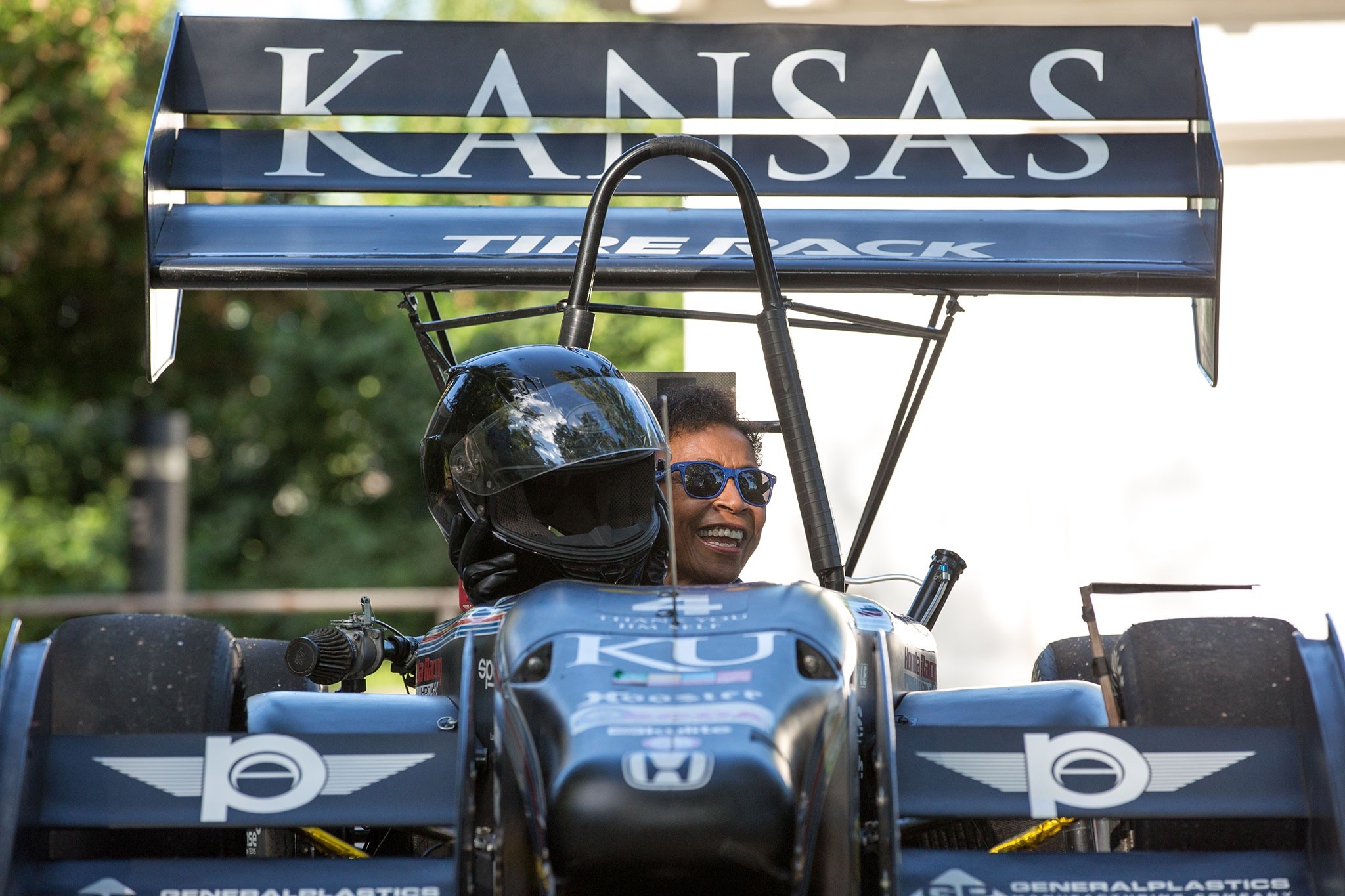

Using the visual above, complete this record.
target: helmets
[419,342,672,601]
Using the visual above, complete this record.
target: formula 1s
[2,9,1345,896]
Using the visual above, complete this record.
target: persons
[447,382,776,608]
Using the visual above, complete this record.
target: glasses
[652,461,776,507]
